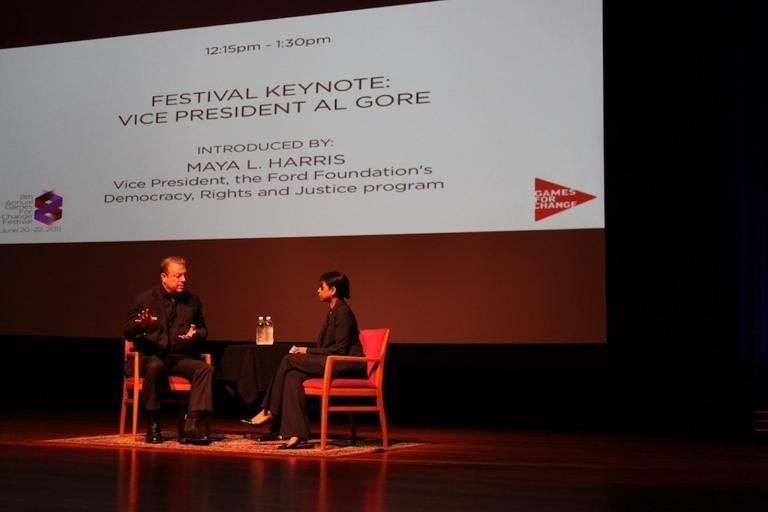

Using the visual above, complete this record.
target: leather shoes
[178,427,209,444]
[146,422,162,443]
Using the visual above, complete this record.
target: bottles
[256,316,266,345]
[265,315,275,345]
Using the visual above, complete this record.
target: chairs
[118,338,212,441]
[302,327,389,450]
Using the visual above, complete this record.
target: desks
[210,340,314,443]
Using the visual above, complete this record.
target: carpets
[46,429,421,457]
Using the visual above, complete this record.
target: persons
[240,272,366,449]
[123,256,215,445]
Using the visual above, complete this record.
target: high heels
[240,414,276,431]
[278,435,309,449]
